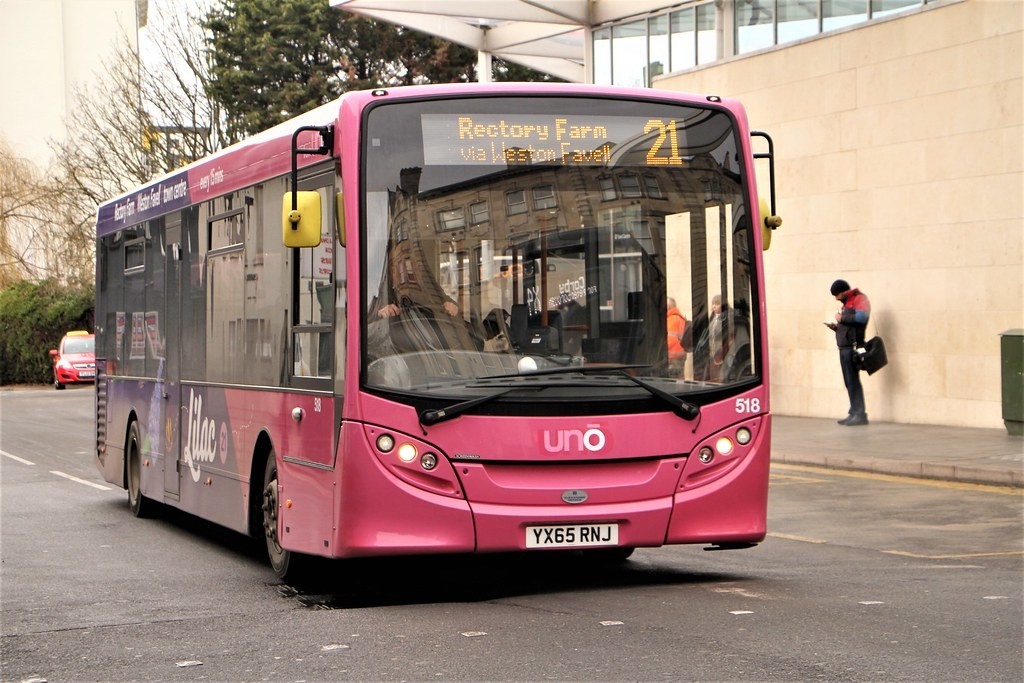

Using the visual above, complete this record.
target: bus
[93,82,783,588]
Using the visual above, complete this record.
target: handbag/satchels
[852,336,888,375]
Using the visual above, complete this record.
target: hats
[830,280,850,296]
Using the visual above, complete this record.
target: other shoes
[847,412,868,426]
[837,413,854,425]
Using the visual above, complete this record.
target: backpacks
[679,320,693,352]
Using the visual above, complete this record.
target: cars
[49,331,113,390]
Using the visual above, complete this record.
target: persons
[364,246,461,327]
[553,290,589,360]
[824,280,871,426]
[666,298,688,379]
[707,295,737,383]
